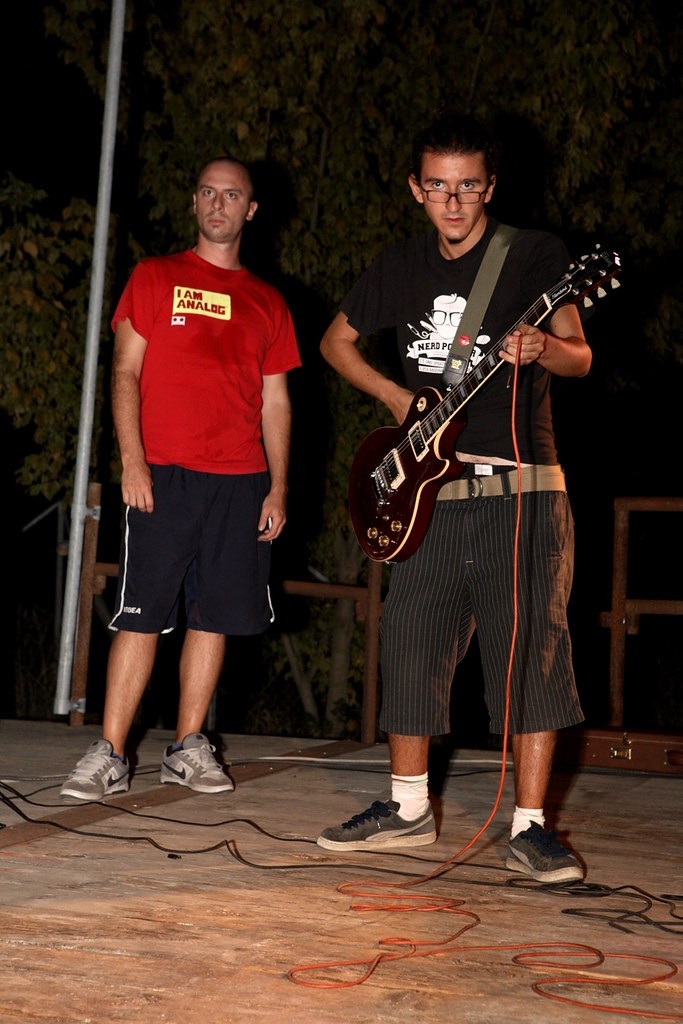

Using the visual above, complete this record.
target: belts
[436,461,567,500]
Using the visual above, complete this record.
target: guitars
[348,236,632,563]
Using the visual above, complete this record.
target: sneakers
[316,799,437,852]
[159,732,235,793]
[59,739,130,803]
[503,822,585,883]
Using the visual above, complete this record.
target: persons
[59,157,303,800]
[320,126,591,883]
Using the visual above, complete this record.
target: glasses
[416,183,489,204]
